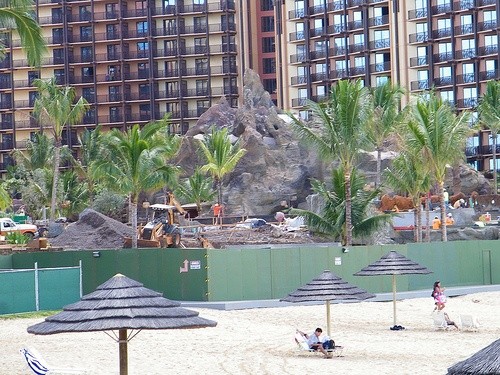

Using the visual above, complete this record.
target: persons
[211,204,222,226]
[296,329,310,345]
[443,188,449,209]
[431,280,445,312]
[445,213,454,226]
[434,299,461,331]
[453,199,468,209]
[448,202,452,206]
[307,328,333,359]
[432,216,440,230]
[271,211,286,224]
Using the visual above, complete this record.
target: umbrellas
[26,273,217,375]
[352,251,434,326]
[279,270,376,341]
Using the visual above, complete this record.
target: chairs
[431,310,453,331]
[20,345,92,375]
[295,338,333,355]
[459,313,482,331]
[301,336,345,355]
